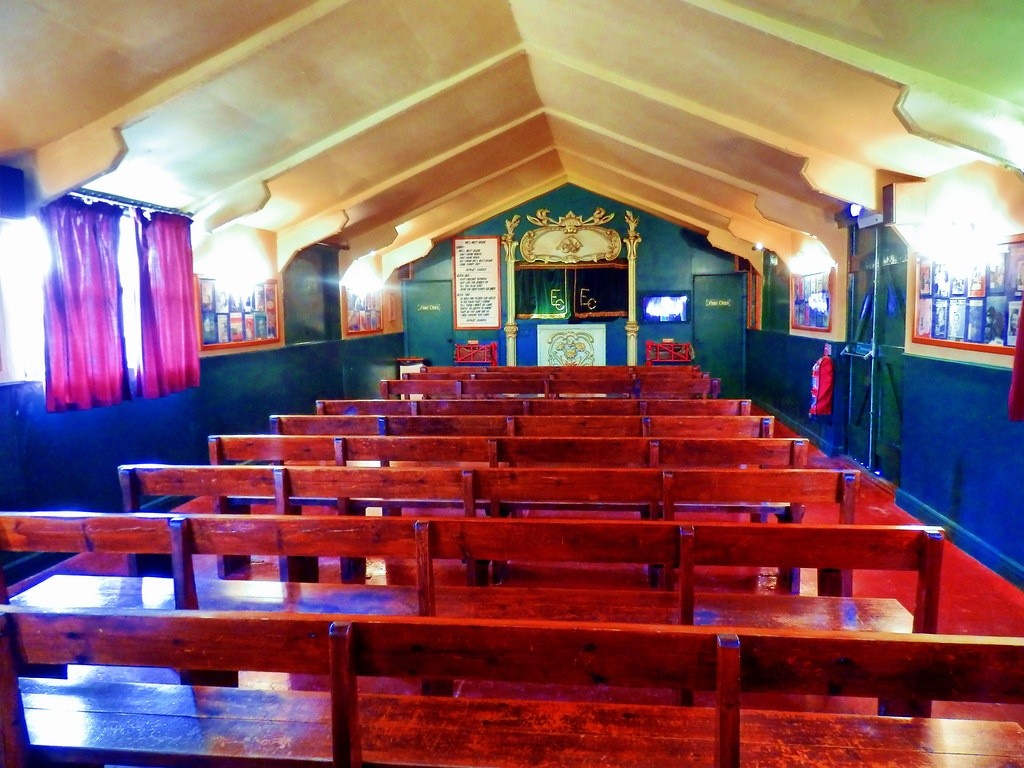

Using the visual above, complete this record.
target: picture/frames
[912,234,1024,356]
[789,266,836,333]
[342,285,383,335]
[194,273,280,351]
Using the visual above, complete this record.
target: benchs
[1,364,1023,768]
